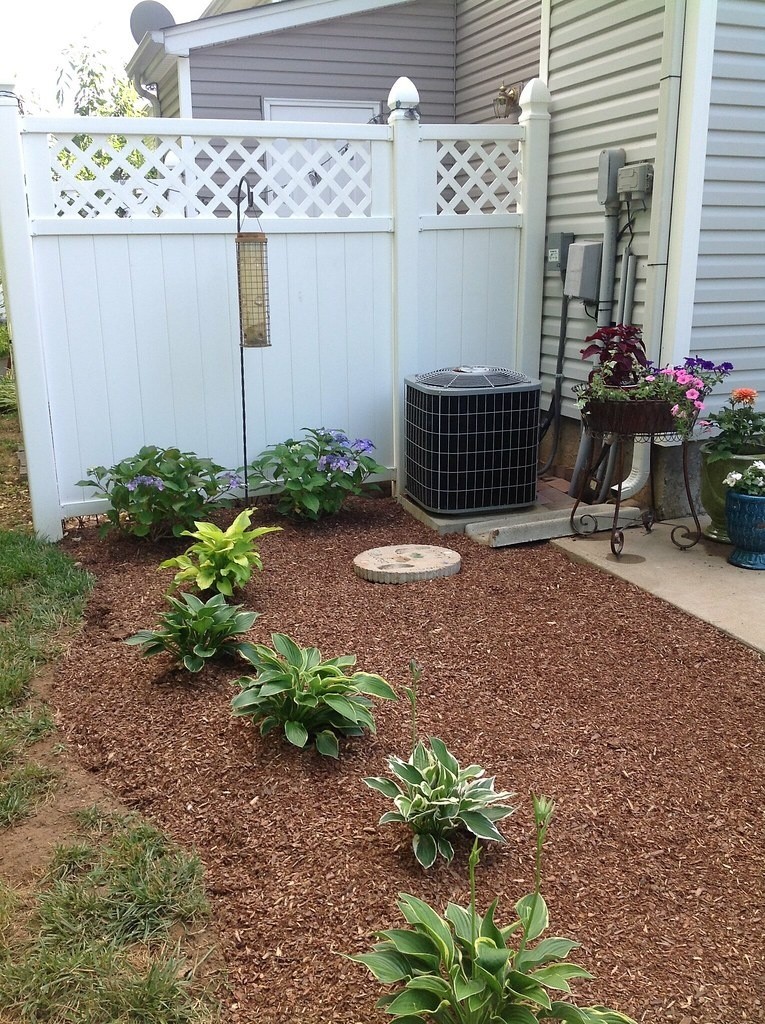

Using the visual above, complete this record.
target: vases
[579,398,703,443]
[699,442,765,543]
[724,488,765,570]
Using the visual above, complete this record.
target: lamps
[493,78,517,119]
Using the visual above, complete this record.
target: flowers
[722,460,765,496]
[699,388,765,463]
[572,322,734,439]
